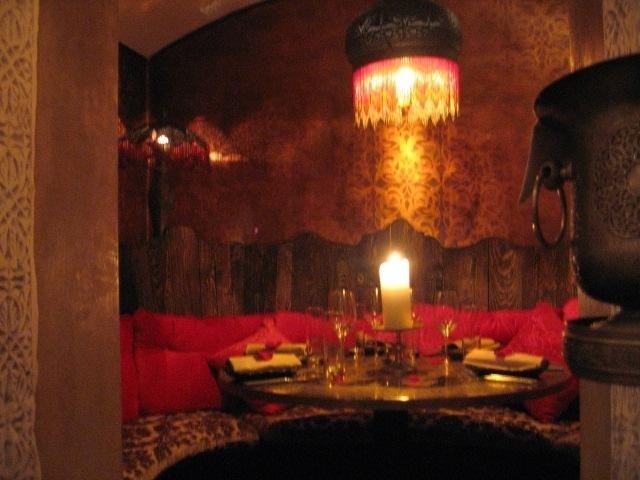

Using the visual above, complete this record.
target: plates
[220,359,298,380]
[465,359,549,380]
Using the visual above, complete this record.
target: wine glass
[433,289,461,380]
[328,287,383,381]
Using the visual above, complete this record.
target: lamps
[340,2,464,134]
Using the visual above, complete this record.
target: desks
[224,347,575,480]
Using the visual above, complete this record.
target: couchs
[116,302,582,474]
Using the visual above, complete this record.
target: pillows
[119,351,138,421]
[496,298,583,424]
[141,349,223,412]
[210,321,305,417]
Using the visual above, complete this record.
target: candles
[377,250,417,329]
